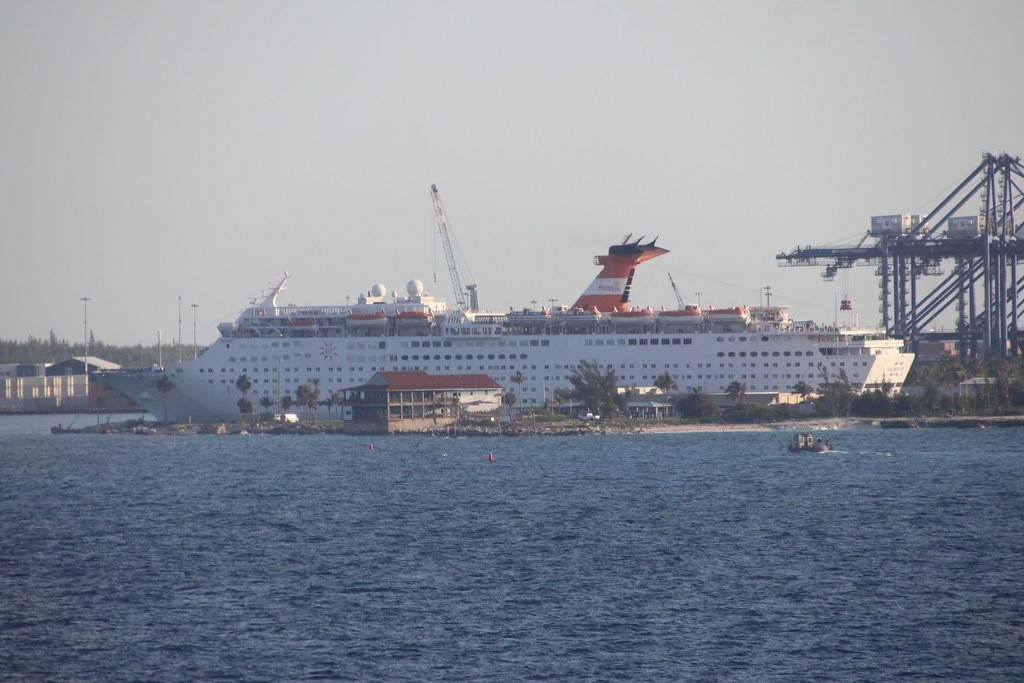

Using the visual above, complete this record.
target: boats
[785,431,833,454]
[395,310,436,329]
[91,233,918,430]
[344,313,388,328]
[609,306,653,325]
[555,305,600,324]
[287,319,320,331]
[505,306,553,326]
[706,307,752,324]
[657,304,702,324]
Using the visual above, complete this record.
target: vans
[272,412,300,425]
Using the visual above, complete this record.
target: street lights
[190,303,200,360]
[80,297,91,397]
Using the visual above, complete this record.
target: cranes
[429,183,479,313]
[667,271,688,312]
[774,150,1024,364]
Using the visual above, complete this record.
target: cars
[579,412,600,421]
